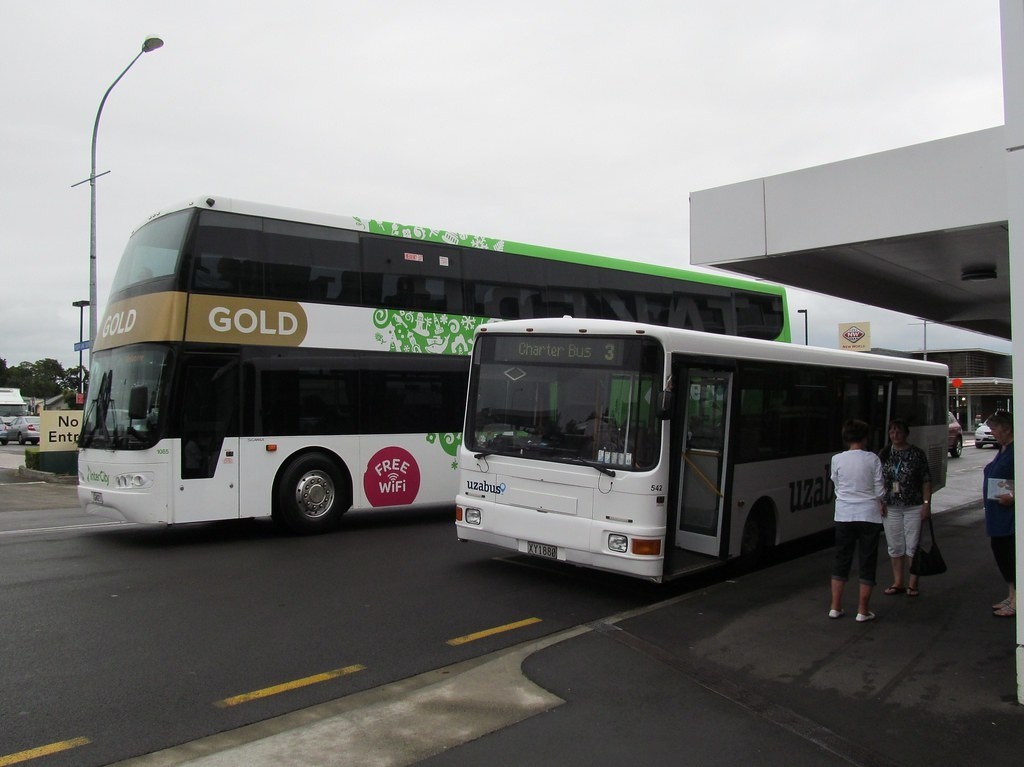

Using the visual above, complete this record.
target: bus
[455,314,950,587]
[75,196,791,538]
[210,355,470,437]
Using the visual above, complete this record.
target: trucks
[0,387,28,445]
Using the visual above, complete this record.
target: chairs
[243,261,783,339]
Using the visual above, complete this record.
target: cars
[948,411,963,458]
[975,416,1002,449]
[0,416,40,444]
[90,409,140,432]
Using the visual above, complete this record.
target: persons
[829,421,887,620]
[983,411,1016,618]
[878,421,934,596]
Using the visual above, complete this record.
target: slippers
[992,597,1011,609]
[907,586,919,596]
[993,605,1016,616]
[885,586,905,594]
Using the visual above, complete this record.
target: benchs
[619,419,646,443]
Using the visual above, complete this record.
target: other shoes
[829,609,845,618]
[856,611,876,621]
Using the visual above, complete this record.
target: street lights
[88,38,164,366]
[798,309,808,346]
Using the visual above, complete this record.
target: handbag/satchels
[909,518,947,576]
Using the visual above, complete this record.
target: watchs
[923,500,930,504]
